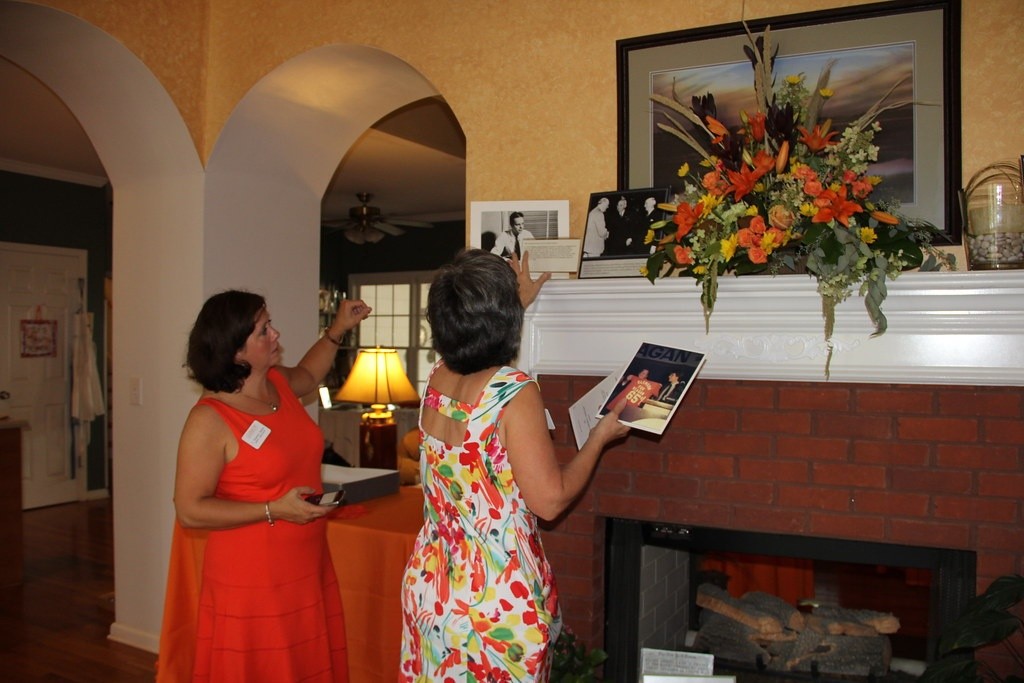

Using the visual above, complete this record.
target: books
[596,340,707,438]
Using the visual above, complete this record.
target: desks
[154,485,424,683]
[643,399,673,416]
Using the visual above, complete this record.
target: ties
[659,384,671,401]
[515,235,521,261]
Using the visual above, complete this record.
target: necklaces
[239,380,278,412]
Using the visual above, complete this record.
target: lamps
[334,343,422,470]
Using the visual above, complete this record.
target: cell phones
[305,490,346,505]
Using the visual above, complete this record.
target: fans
[321,193,434,243]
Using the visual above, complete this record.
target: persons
[489,211,536,260]
[626,198,662,254]
[174,291,373,683]
[605,199,635,255]
[398,249,632,683]
[604,368,684,418]
[582,197,610,257]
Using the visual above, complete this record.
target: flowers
[641,0,957,382]
[549,622,610,683]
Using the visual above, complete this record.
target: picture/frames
[616,0,962,245]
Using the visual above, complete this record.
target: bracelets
[265,500,275,527]
[324,329,344,345]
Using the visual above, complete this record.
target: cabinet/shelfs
[0,419,33,589]
